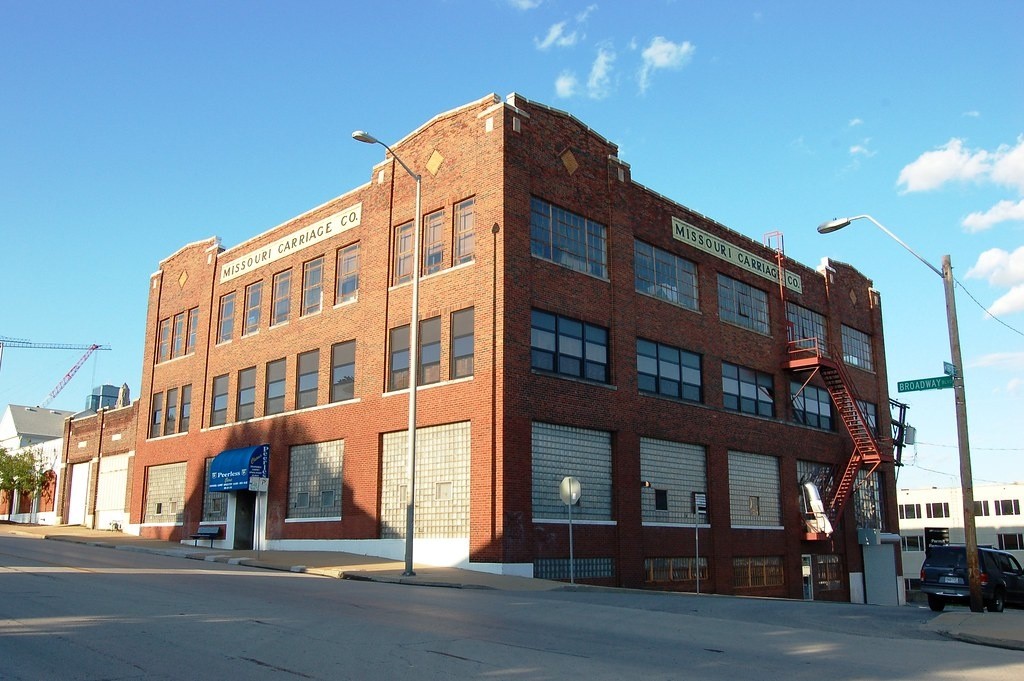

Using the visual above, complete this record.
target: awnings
[208,445,270,492]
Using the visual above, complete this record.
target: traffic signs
[897,376,955,394]
[943,361,955,377]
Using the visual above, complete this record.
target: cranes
[37,342,101,409]
[0,334,112,375]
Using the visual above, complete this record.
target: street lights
[816,212,985,612]
[353,130,422,579]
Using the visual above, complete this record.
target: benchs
[189,526,221,548]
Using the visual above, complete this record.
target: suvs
[920,544,1024,614]
